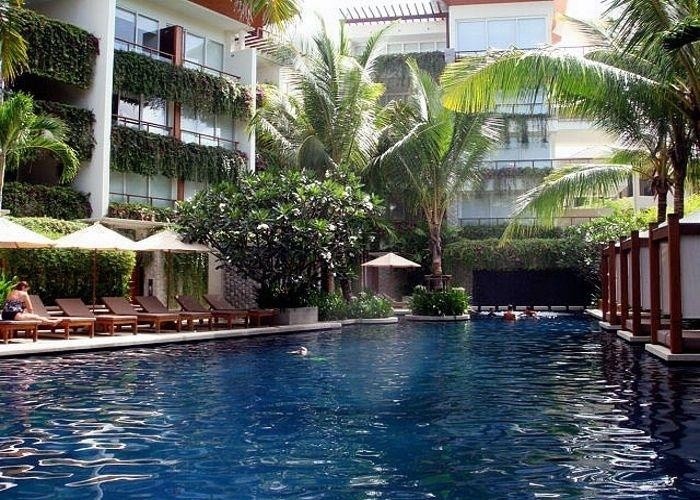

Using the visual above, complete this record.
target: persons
[2,281,63,327]
[488,305,537,322]
[285,346,321,362]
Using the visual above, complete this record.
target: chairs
[0,292,276,344]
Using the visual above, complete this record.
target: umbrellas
[360,252,421,298]
[52,221,210,316]
[0,216,53,247]
